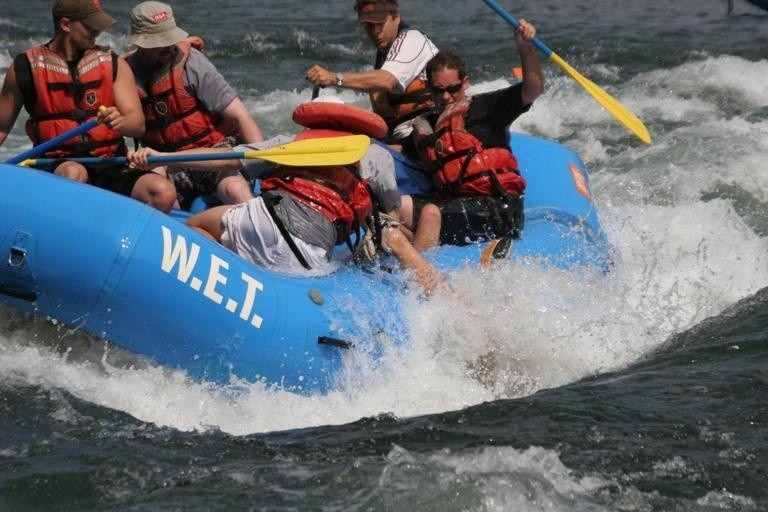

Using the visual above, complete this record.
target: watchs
[336,71,343,89]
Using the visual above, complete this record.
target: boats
[1,132,611,396]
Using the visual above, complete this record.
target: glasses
[428,80,463,94]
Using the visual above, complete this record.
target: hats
[353,1,399,25]
[123,0,190,50]
[52,0,119,32]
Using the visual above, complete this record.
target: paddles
[15,134,372,170]
[486,0,655,145]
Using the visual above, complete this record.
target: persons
[125,102,454,304]
[119,2,263,212]
[382,19,545,255]
[306,1,440,162]
[0,1,177,213]
